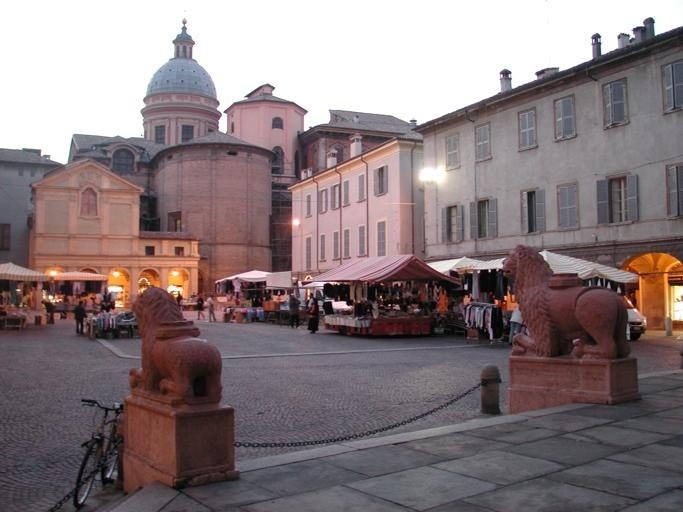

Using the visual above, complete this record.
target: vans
[621,294,648,342]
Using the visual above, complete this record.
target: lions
[502,245,630,359]
[129,287,221,399]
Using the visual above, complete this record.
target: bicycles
[73,399,124,508]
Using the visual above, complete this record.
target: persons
[176,291,183,308]
[308,299,319,334]
[197,292,205,320]
[41,292,115,336]
[289,292,301,328]
[208,294,217,322]
[308,293,315,310]
